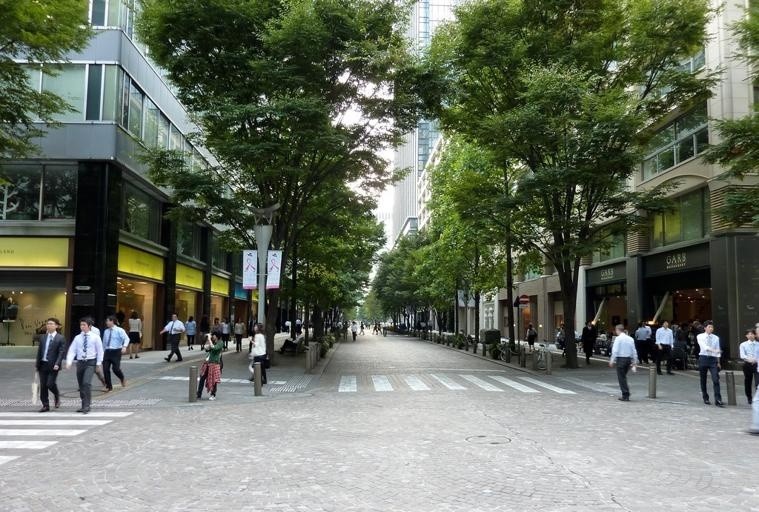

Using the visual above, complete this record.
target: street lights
[246,203,285,333]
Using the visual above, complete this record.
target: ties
[46,335,52,360]
[107,329,112,347]
[82,335,88,363]
[169,321,175,335]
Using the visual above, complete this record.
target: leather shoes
[657,372,664,375]
[715,400,725,407]
[704,399,710,404]
[75,407,91,414]
[164,358,170,362]
[54,398,60,408]
[38,406,50,412]
[175,358,183,362]
[618,392,630,401]
[666,371,675,375]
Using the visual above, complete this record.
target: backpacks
[220,355,223,374]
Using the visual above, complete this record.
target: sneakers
[129,356,139,359]
[102,384,112,392]
[121,377,128,387]
[249,374,267,386]
[208,395,216,401]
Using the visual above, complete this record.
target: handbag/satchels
[265,354,272,369]
[31,383,40,406]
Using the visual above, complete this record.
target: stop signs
[519,294,529,305]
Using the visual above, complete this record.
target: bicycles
[457,330,551,370]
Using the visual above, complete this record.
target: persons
[753,322,759,388]
[196,331,224,401]
[6,297,17,320]
[66,317,104,412]
[36,319,66,411]
[101,315,131,392]
[283,317,433,342]
[738,328,759,404]
[85,318,106,386]
[556,319,705,375]
[525,322,538,352]
[114,309,268,386]
[0,293,7,323]
[696,320,725,407]
[609,325,638,401]
